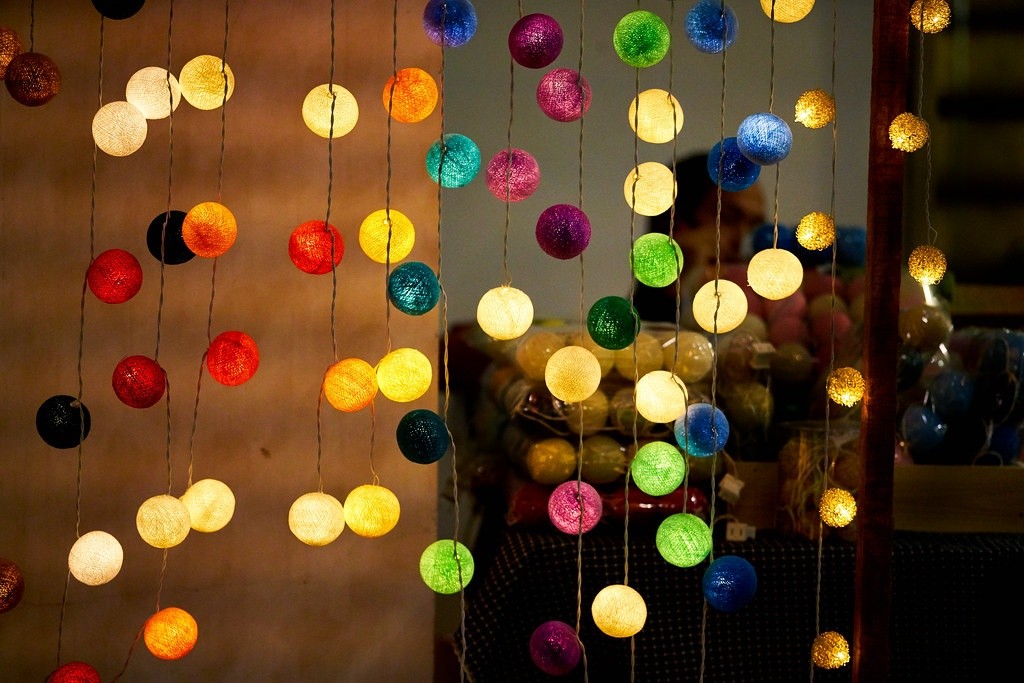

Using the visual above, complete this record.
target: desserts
[459,270,1024,489]
